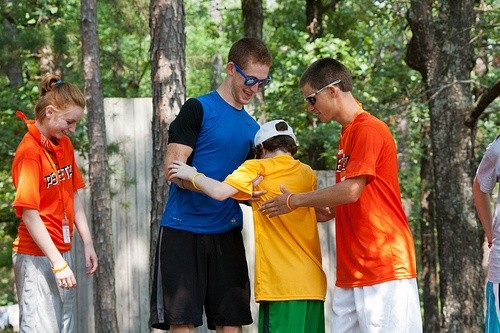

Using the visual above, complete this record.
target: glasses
[304,80,341,105]
[235,64,271,88]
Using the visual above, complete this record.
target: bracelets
[51,263,68,273]
[192,173,206,190]
[286,193,295,211]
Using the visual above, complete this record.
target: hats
[254,119,297,148]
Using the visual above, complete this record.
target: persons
[12,74,98,333]
[150,37,272,333]
[257,57,423,332]
[167,119,327,333]
[473,139,500,333]
